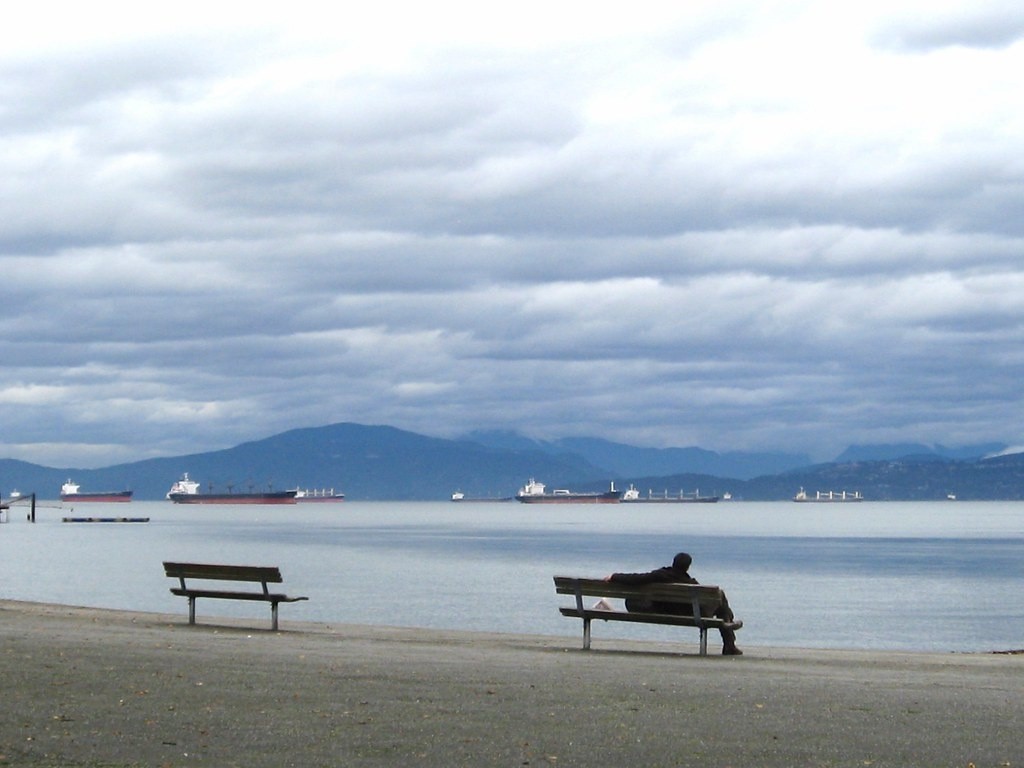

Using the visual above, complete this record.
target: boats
[791,486,865,503]
[165,473,346,505]
[59,478,134,504]
[452,488,513,503]
[618,487,721,506]
[513,477,623,505]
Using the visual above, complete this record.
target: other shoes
[730,619,743,629]
[722,644,743,655]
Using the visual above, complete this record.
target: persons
[603,552,745,656]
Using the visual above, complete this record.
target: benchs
[163,560,309,629]
[554,575,738,657]
